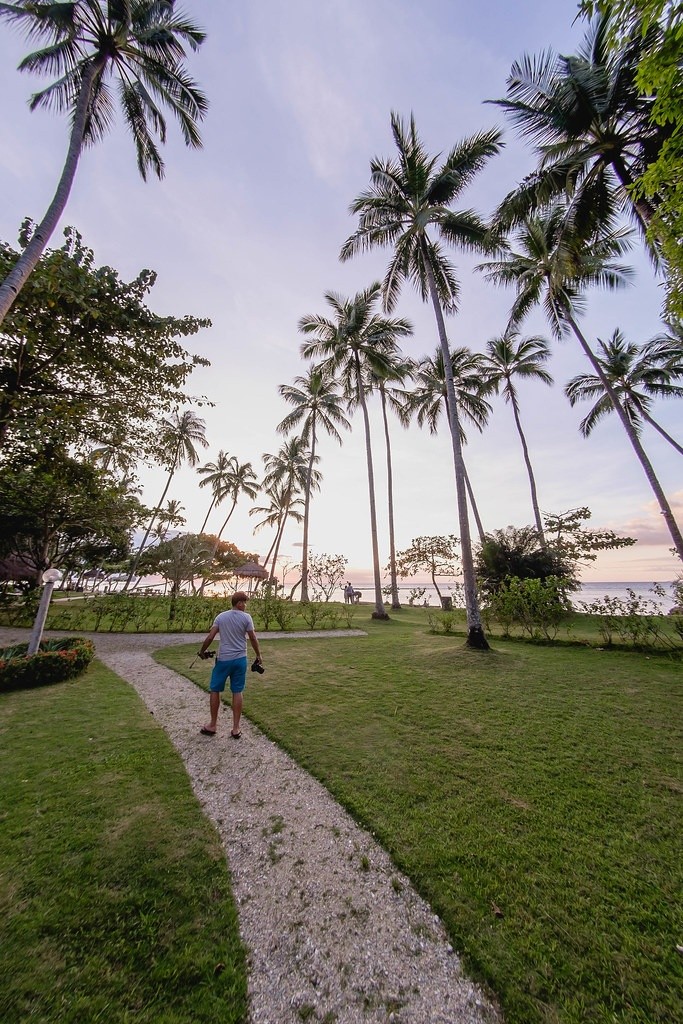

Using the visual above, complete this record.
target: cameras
[251,658,265,674]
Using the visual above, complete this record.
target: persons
[200,593,262,738]
[342,583,354,604]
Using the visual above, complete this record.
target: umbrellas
[83,570,104,579]
[233,562,269,597]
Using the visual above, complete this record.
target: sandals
[200,726,217,736]
[231,729,242,739]
